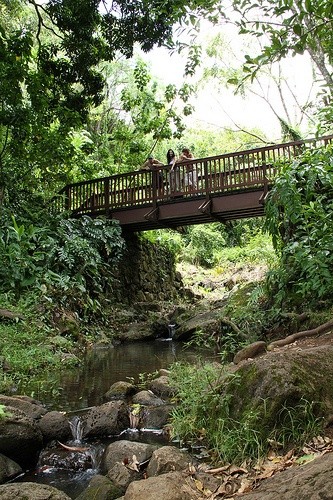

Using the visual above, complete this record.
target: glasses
[148,158,153,159]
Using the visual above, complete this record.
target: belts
[186,169,195,173]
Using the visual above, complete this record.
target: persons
[167,149,185,200]
[176,148,201,198]
[139,154,166,201]
